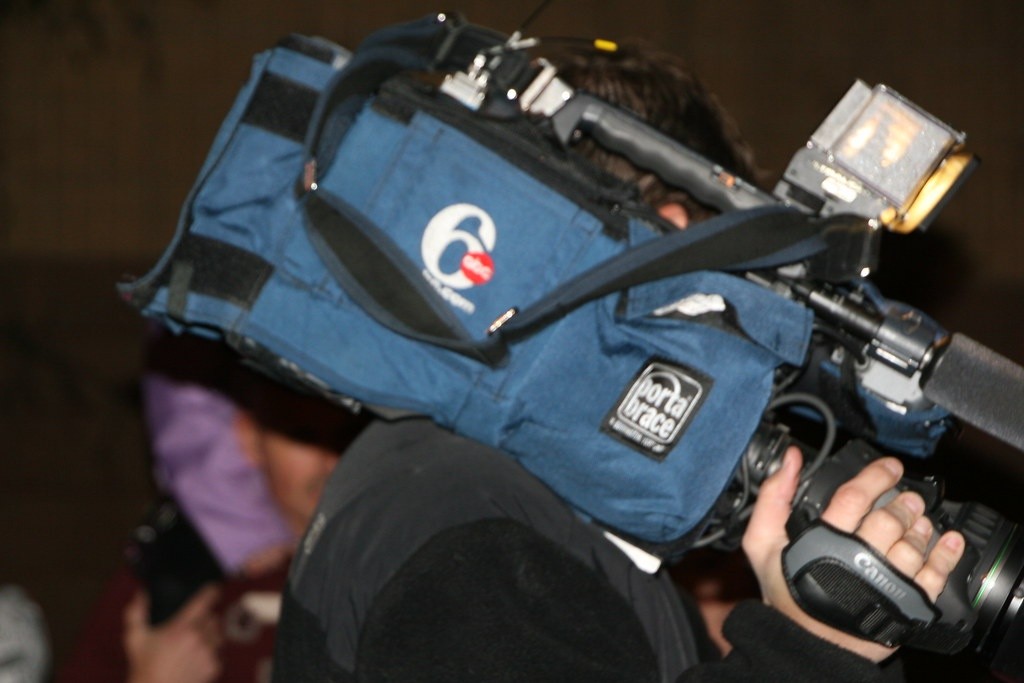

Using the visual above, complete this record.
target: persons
[274,46,967,682]
[46,322,373,683]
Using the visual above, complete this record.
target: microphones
[793,278,1024,451]
